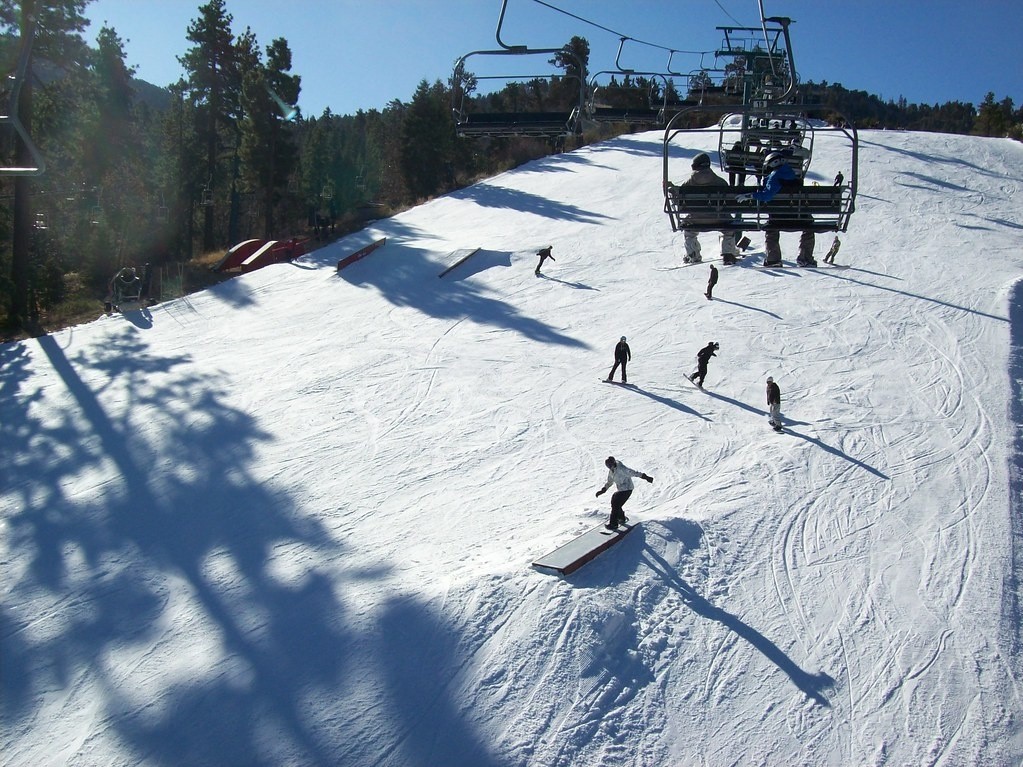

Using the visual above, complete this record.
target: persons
[667,152,741,265]
[704,264,718,299]
[756,149,769,187]
[834,171,844,186]
[735,151,817,268]
[766,376,782,432]
[770,121,784,145]
[691,341,720,388]
[595,456,654,531]
[285,239,294,262]
[823,236,841,263]
[605,336,632,385]
[535,246,555,271]
[729,141,746,187]
[787,120,797,129]
[142,263,152,293]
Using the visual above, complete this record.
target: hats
[767,376,773,382]
[621,336,626,340]
[713,341,719,348]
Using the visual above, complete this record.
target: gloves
[640,474,653,484]
[596,487,607,498]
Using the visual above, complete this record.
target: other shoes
[773,426,781,431]
[690,373,695,381]
[618,518,625,525]
[622,381,626,384]
[770,422,776,426]
[697,382,703,389]
[605,523,618,530]
[606,379,612,382]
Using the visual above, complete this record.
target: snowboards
[683,372,707,391]
[599,516,630,535]
[741,263,852,271]
[704,292,713,301]
[768,420,784,434]
[823,259,838,266]
[534,270,542,277]
[601,379,634,386]
[649,249,766,271]
